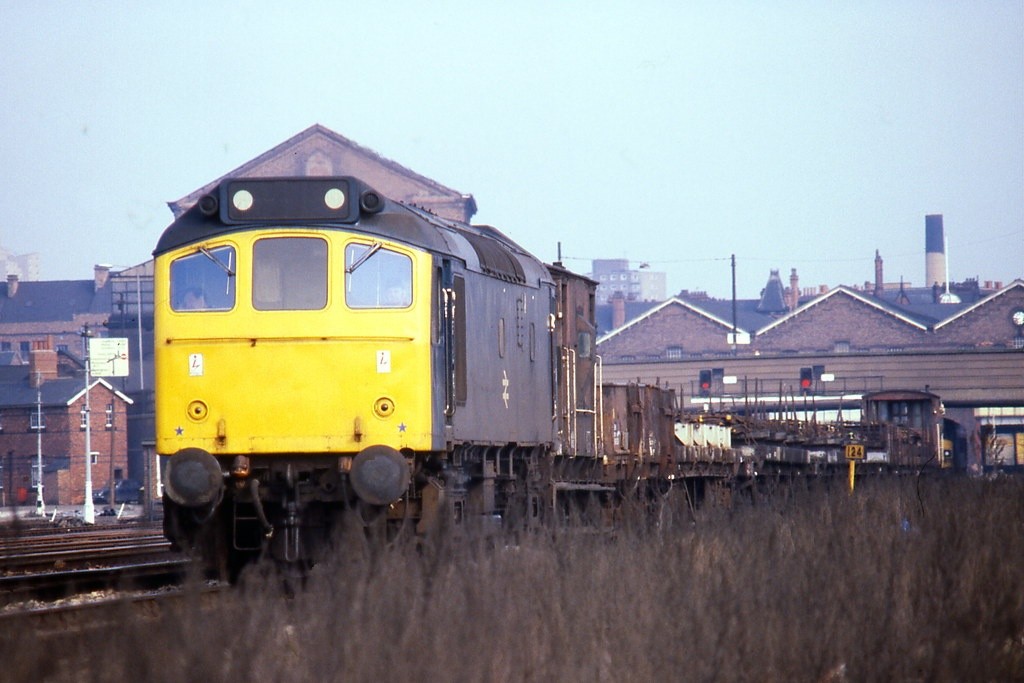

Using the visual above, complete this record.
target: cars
[92,479,140,504]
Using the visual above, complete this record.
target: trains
[151,175,1023,583]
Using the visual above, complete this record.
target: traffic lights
[800,368,812,388]
[699,369,711,397]
